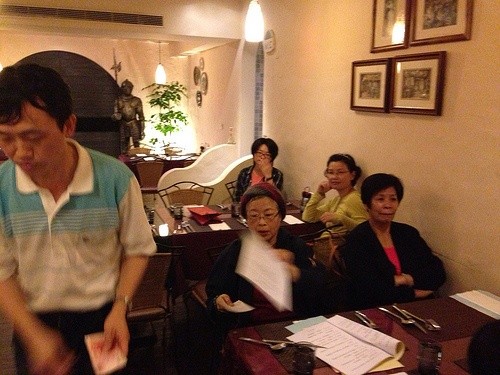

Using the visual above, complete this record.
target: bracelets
[115,296,132,312]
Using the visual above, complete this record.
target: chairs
[184,223,352,375]
[135,155,163,187]
[158,181,215,208]
[126,253,178,375]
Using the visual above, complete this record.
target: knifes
[392,305,427,333]
[262,339,326,348]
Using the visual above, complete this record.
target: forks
[402,310,440,331]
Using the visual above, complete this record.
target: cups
[174,203,183,220]
[145,210,155,225]
[418,338,442,375]
[295,341,316,375]
[231,202,240,217]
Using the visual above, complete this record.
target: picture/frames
[370,0,409,52]
[350,57,390,113]
[388,50,446,116]
[193,57,207,106]
[409,0,473,45]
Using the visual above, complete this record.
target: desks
[228,296,495,375]
[156,201,307,236]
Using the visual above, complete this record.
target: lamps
[244,0,265,43]
[155,41,166,84]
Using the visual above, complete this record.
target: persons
[205,183,319,331]
[234,138,283,201]
[0,64,157,375]
[302,153,368,238]
[342,173,447,308]
[112,80,145,155]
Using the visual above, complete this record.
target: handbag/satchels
[187,205,223,224]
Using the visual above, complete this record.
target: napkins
[458,291,500,316]
[282,215,305,225]
[159,223,169,237]
[231,300,256,312]
[209,222,231,231]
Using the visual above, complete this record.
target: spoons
[355,311,379,328]
[379,308,415,324]
[239,337,286,350]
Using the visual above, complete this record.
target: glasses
[245,211,279,225]
[256,152,271,159]
